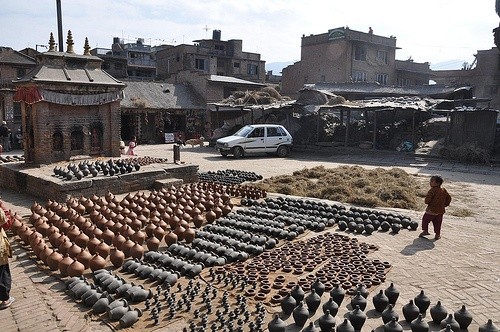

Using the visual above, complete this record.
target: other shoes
[435,234,442,240]
[420,231,430,236]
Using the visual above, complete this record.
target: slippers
[0,297,15,308]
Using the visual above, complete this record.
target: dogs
[120,141,126,155]
[126,142,135,155]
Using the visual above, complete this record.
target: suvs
[215,124,294,159]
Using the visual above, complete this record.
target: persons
[8,128,14,149]
[120,138,125,155]
[15,128,24,148]
[91,129,100,147]
[0,121,10,152]
[419,175,451,239]
[0,200,16,308]
[126,135,137,155]
